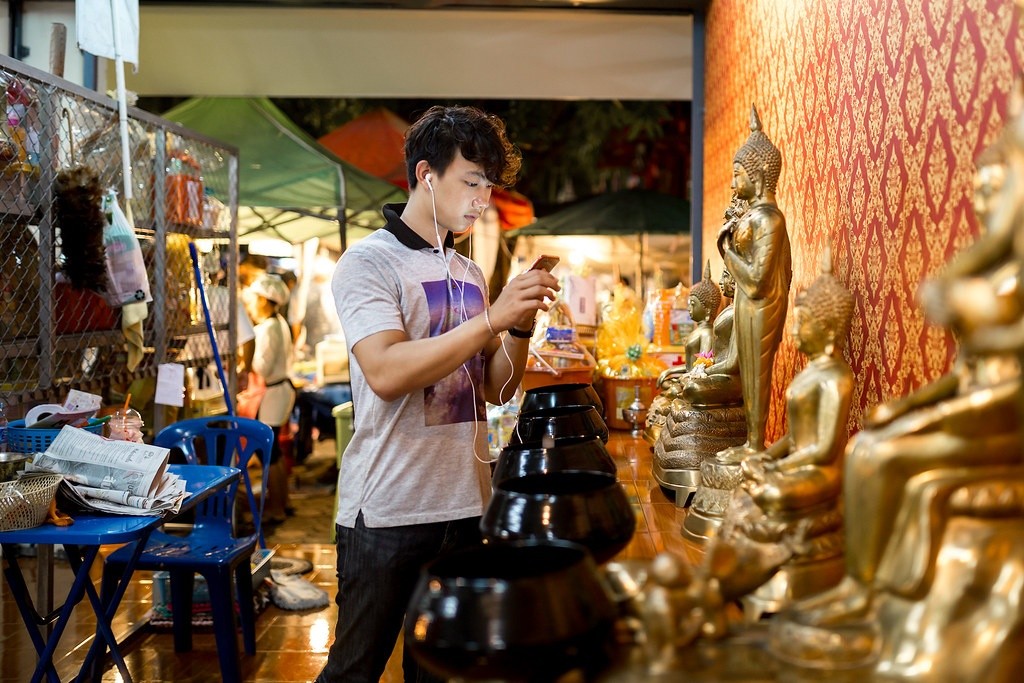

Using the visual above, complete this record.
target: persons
[186,253,303,533]
[793,114,1023,628]
[315,106,560,683]
[717,130,790,464]
[741,273,854,515]
[656,281,721,398]
[677,266,741,404]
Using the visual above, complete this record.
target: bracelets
[485,307,495,336]
[508,319,537,338]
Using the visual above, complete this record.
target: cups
[108,408,144,441]
[203,204,221,230]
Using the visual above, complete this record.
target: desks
[0,464,242,683]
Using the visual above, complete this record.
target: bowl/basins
[0,451,28,481]
[403,540,615,683]
[491,435,617,489]
[520,383,604,418]
[478,469,635,570]
[509,404,607,445]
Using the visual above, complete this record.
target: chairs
[91,416,274,683]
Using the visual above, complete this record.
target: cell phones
[520,255,559,275]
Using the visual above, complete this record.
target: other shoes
[272,506,294,525]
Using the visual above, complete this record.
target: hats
[203,255,224,280]
[249,273,290,305]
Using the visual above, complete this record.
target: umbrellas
[523,187,691,296]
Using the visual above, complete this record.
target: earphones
[425,174,433,192]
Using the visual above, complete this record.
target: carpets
[270,558,314,575]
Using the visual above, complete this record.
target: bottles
[0,402,9,451]
[102,194,146,305]
[150,133,203,226]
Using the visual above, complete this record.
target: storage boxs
[599,373,662,430]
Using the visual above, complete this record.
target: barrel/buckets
[315,333,350,388]
[314,381,351,440]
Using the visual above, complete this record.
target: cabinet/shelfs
[0,54,240,681]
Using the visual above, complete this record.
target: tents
[157,98,406,254]
[317,107,534,230]
